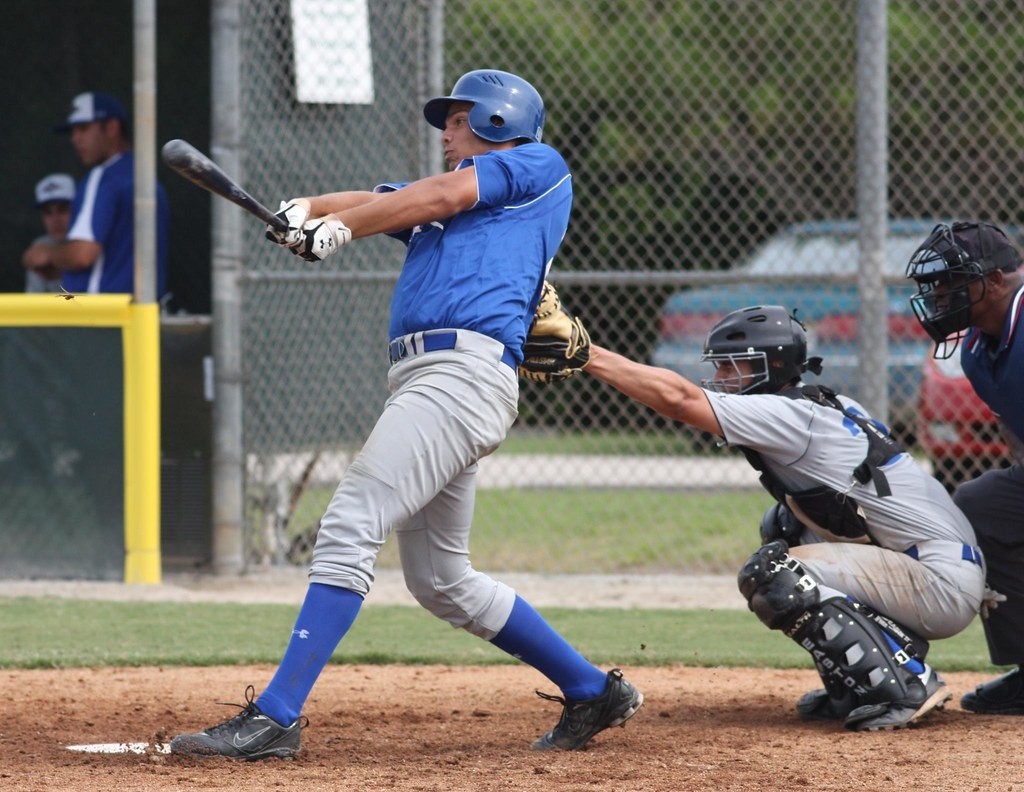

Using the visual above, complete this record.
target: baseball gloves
[515,275,592,383]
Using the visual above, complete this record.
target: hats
[66,91,132,126]
[35,171,78,206]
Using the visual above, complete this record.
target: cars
[646,215,1023,495]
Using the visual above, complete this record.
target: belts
[387,332,517,372]
[901,543,984,570]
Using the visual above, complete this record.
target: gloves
[265,198,311,248]
[287,213,353,262]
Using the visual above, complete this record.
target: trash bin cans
[163,318,212,566]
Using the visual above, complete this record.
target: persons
[24,90,171,308]
[170,68,643,758]
[905,221,1024,714]
[516,281,988,733]
[25,172,80,293]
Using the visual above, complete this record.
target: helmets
[704,305,808,374]
[913,220,1021,288]
[423,69,544,144]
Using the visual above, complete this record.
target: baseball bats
[159,139,289,233]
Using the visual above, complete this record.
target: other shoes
[796,686,839,721]
[855,662,954,731]
[961,668,1024,715]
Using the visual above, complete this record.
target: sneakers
[170,685,308,763]
[529,669,646,752]
